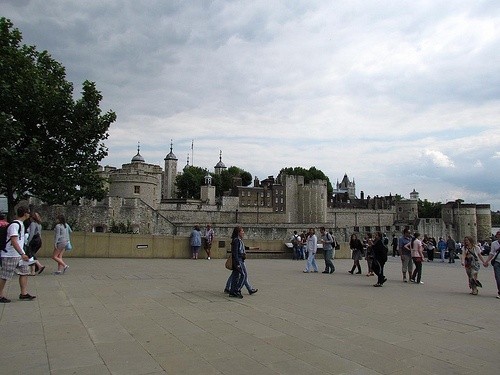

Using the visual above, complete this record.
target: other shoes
[304,270,307,273]
[0,297,11,303]
[367,272,374,276]
[224,287,258,298]
[27,272,35,276]
[20,294,36,299]
[53,271,61,274]
[470,288,478,294]
[496,294,500,299]
[374,279,387,287]
[38,266,45,274]
[403,278,424,284]
[322,271,329,273]
[330,269,335,274]
[63,265,70,272]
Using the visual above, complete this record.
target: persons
[291,225,341,275]
[0,209,45,276]
[0,207,36,303]
[202,225,215,261]
[347,231,500,298]
[50,214,73,276]
[223,224,259,299]
[190,225,202,260]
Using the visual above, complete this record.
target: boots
[356,265,361,274]
[348,265,356,274]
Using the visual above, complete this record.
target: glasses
[26,212,30,214]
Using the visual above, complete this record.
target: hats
[33,213,41,223]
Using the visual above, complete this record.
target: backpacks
[0,220,20,250]
[293,235,299,247]
[327,232,335,248]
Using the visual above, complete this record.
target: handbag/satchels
[491,259,495,266]
[66,241,72,250]
[225,256,233,270]
[29,234,41,248]
